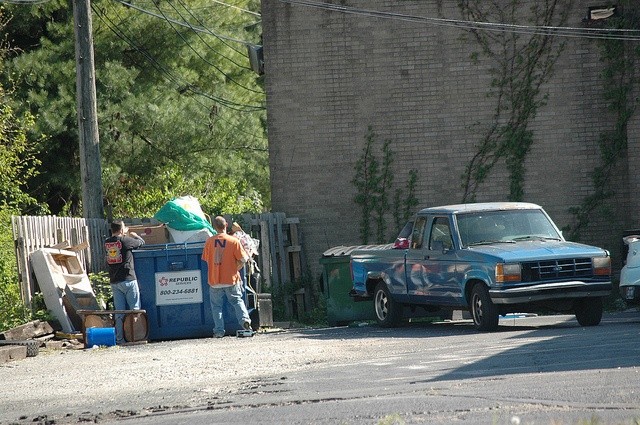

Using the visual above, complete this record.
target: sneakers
[243,321,253,330]
[214,331,224,337]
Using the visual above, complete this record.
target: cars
[618,235,640,306]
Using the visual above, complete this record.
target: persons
[103,219,145,348]
[201,215,252,339]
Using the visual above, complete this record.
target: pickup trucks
[350,202,613,331]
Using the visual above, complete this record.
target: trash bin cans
[130,241,244,342]
[316,243,394,326]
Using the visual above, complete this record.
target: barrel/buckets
[86,328,116,348]
